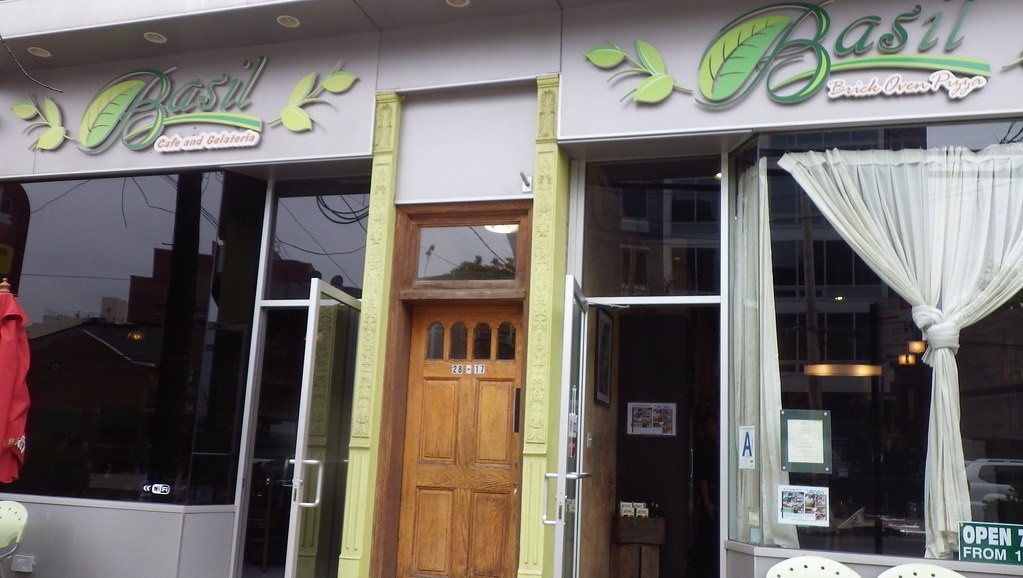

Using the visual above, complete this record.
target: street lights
[869,303,926,553]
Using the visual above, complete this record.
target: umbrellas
[0,278,31,483]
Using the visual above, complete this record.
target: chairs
[875,563,968,578]
[243,462,286,571]
[0,501,28,562]
[766,556,861,578]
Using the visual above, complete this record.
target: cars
[142,455,350,566]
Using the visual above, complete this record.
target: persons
[698,410,718,578]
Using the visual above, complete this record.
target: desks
[89,471,169,502]
[611,515,666,578]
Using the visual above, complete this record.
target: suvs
[964,458,1023,526]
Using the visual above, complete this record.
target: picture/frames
[593,306,614,409]
